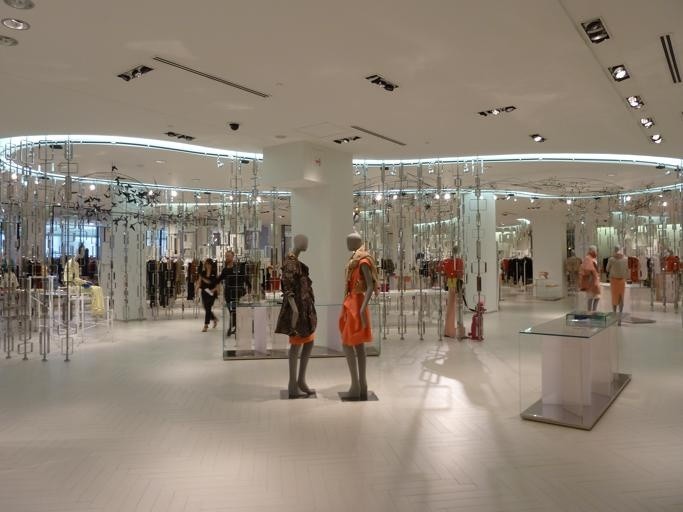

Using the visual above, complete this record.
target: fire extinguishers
[471,304,487,341]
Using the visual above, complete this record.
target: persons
[207,249,251,337]
[577,244,601,311]
[271,233,316,397]
[193,258,219,333]
[605,242,629,327]
[442,245,463,340]
[61,243,105,316]
[338,234,375,402]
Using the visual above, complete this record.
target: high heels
[201,318,219,332]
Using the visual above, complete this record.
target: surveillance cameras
[229,124,239,130]
